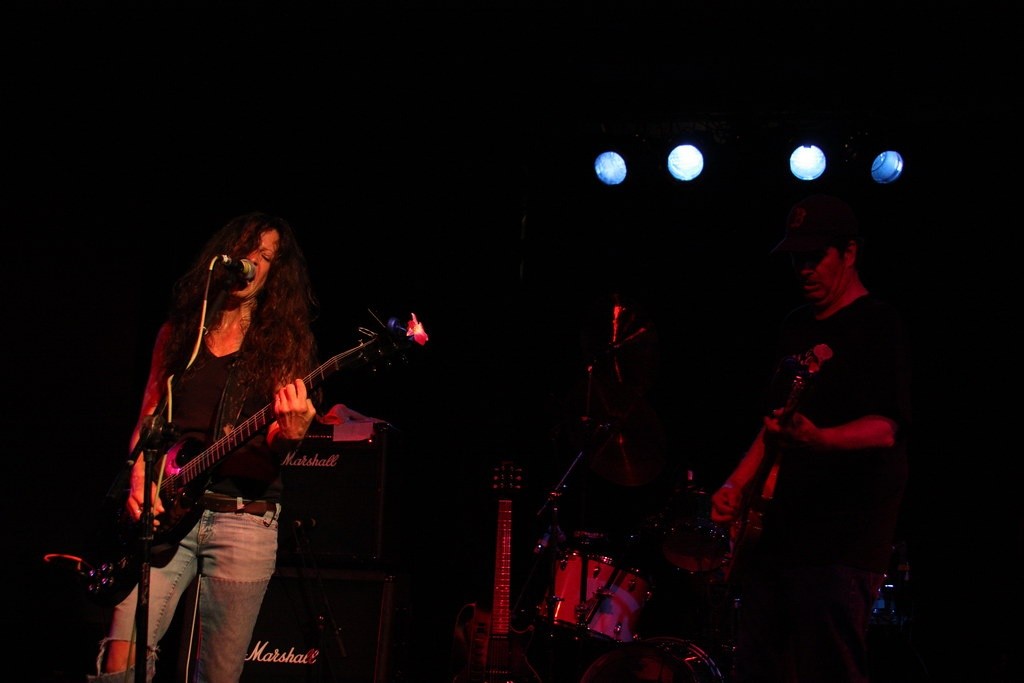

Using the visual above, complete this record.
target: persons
[711,206,919,683]
[87,214,326,682]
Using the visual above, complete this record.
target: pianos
[239,412,413,683]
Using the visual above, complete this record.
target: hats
[771,195,868,253]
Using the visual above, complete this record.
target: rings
[134,509,140,516]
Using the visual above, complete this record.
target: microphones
[218,254,256,281]
[293,518,316,527]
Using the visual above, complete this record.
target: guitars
[717,342,834,587]
[70,312,428,610]
[586,304,672,486]
[447,459,543,683]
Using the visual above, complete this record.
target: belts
[202,496,277,514]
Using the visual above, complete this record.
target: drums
[579,637,724,683]
[538,546,651,648]
[660,490,730,571]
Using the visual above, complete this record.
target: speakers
[177,568,395,683]
[274,432,392,569]
